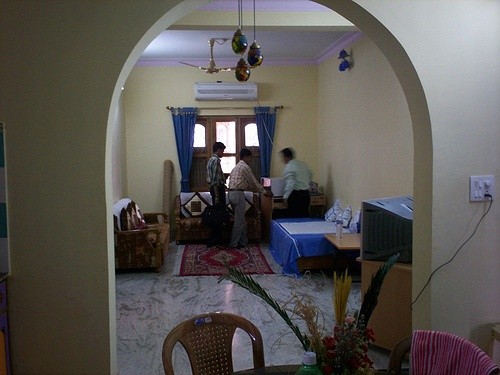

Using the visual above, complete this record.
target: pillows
[325,201,360,233]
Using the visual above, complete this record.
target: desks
[362,261,412,356]
[261,195,324,238]
[323,234,362,283]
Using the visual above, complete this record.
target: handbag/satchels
[202,206,229,225]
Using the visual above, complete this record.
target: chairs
[161,310,264,375]
[387,330,500,374]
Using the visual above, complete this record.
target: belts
[227,188,244,191]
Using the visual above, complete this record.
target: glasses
[249,156,253,159]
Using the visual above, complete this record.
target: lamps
[336,50,351,71]
[231,1,261,82]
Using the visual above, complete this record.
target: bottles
[295,351,323,375]
[336,215,343,241]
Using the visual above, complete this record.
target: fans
[178,40,256,72]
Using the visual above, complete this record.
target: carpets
[180,243,275,277]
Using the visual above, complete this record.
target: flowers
[217,252,401,375]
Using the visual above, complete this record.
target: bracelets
[214,198,220,199]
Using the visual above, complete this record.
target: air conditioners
[194,82,259,101]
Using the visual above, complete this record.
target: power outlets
[470,175,494,202]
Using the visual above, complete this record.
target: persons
[279,148,311,218]
[227,147,272,250]
[206,142,230,248]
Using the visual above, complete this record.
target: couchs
[176,191,261,243]
[113,198,169,270]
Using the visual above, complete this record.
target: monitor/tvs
[361,195,413,264]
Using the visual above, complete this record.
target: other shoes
[206,239,227,247]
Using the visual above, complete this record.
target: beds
[269,216,361,277]
